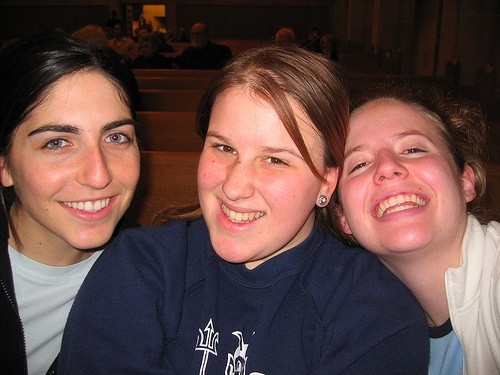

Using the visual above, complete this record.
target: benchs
[126,40,500,227]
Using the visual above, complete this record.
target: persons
[129,18,153,40]
[315,76,500,375]
[0,29,143,375]
[58,42,429,375]
[106,20,135,54]
[71,24,111,70]
[131,34,185,69]
[301,27,322,53]
[318,33,337,61]
[175,23,234,69]
[274,28,296,48]
